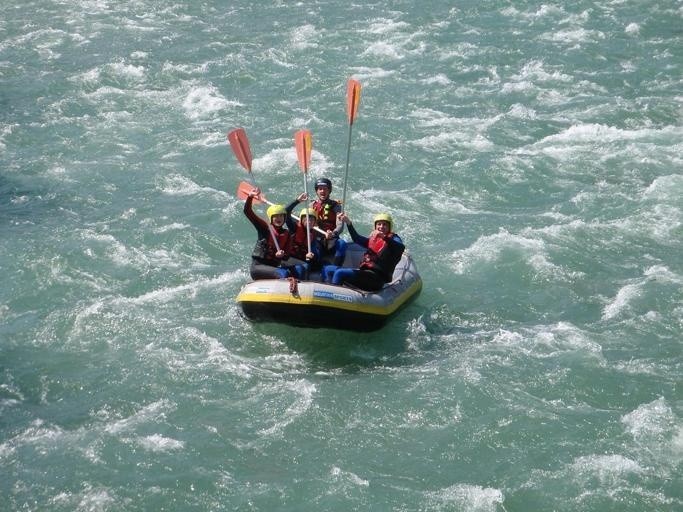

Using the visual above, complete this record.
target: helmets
[314,178,332,193]
[374,213,393,232]
[267,205,288,224]
[299,208,317,226]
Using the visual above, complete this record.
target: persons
[244,187,305,279]
[308,178,346,272]
[281,192,320,280]
[322,213,405,292]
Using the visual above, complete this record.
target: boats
[234,247,427,336]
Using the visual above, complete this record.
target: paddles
[295,130,312,253]
[228,128,280,250]
[341,78,361,214]
[237,181,328,235]
